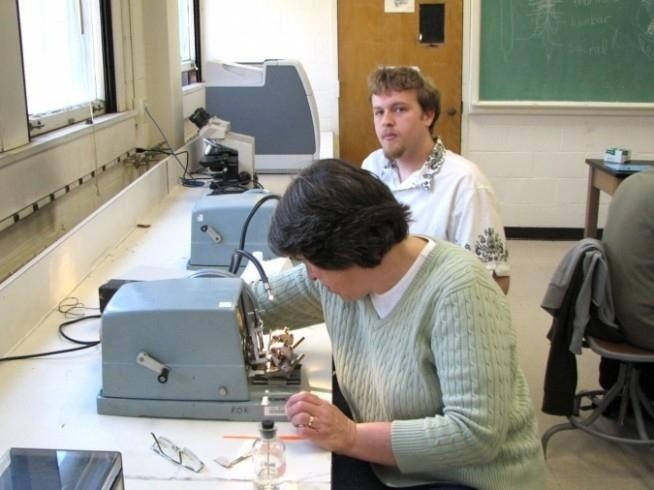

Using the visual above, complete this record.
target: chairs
[540,237,654,461]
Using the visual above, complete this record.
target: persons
[599,168,653,418]
[248,160,549,490]
[361,66,512,296]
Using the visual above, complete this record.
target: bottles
[251,422,288,489]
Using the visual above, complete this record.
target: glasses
[150,430,206,473]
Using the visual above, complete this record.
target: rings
[308,416,314,427]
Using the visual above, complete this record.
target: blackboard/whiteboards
[470,0,654,108]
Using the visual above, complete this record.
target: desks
[0,131,333,490]
[578,159,654,239]
